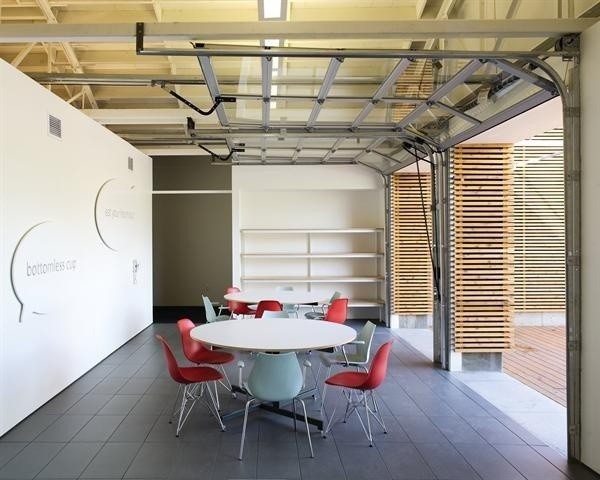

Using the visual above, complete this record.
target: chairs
[157,286,394,461]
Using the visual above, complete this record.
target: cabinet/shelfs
[239,228,385,322]
